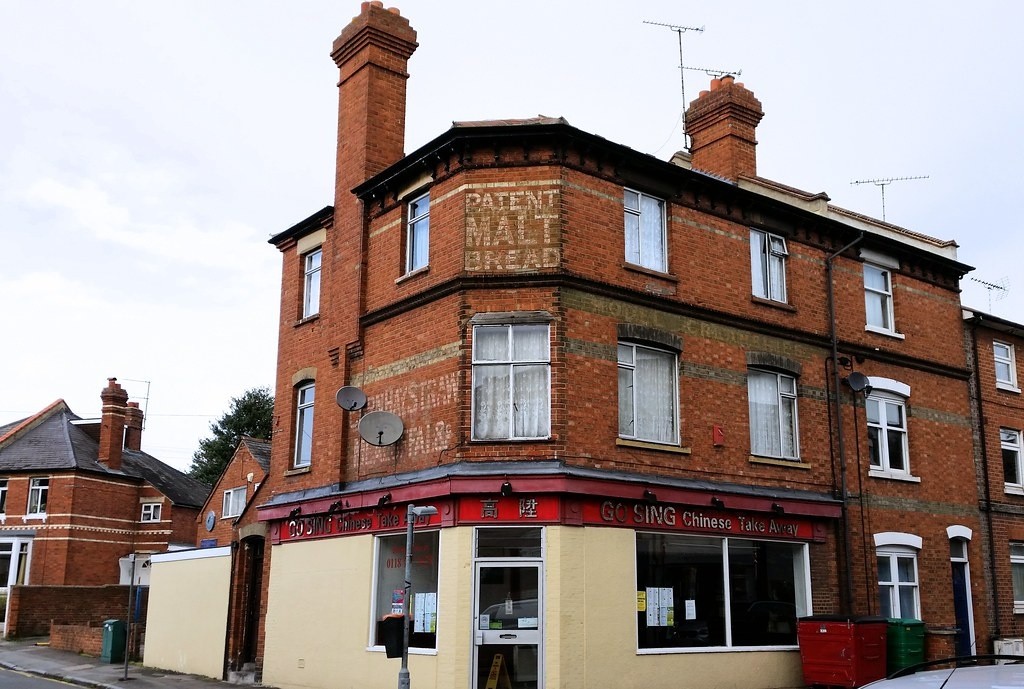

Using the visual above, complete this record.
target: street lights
[397,504,440,689]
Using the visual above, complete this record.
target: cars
[478,598,538,629]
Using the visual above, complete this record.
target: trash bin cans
[101,619,126,663]
[799,616,888,688]
[889,618,926,671]
